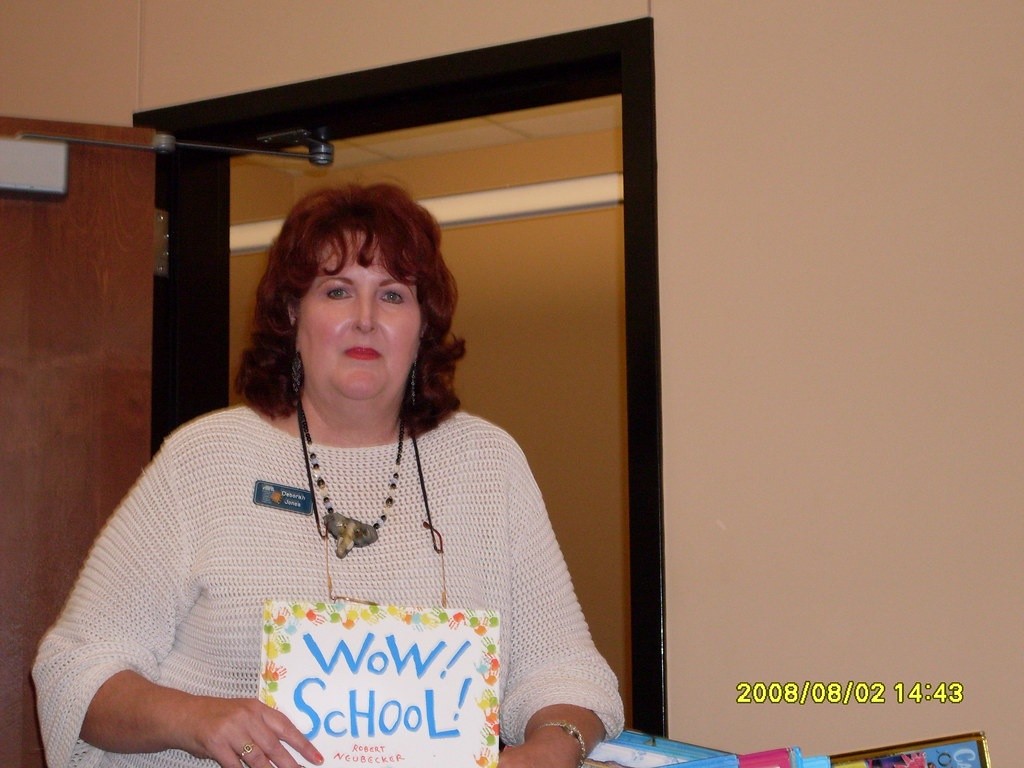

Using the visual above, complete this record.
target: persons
[28,182,625,765]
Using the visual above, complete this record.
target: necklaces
[301,403,405,559]
[295,394,447,610]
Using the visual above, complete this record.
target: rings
[240,743,255,757]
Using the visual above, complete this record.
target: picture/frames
[830,730,991,768]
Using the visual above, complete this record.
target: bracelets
[533,721,586,768]
